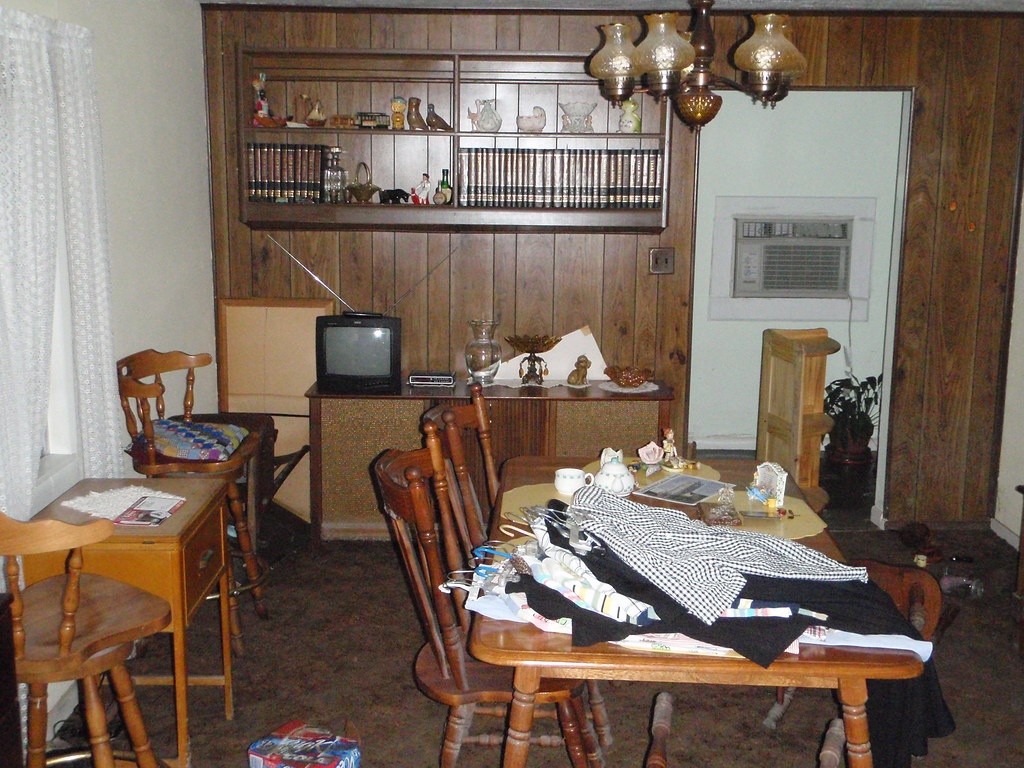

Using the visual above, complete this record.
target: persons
[253,81,288,128]
[662,428,687,468]
[411,173,432,204]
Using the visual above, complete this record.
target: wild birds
[407,97,429,131]
[427,103,452,131]
[466,98,484,131]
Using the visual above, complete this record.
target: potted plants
[818,365,883,459]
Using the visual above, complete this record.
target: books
[112,497,186,526]
[247,143,322,204]
[458,148,665,208]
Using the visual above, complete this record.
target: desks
[467,452,927,768]
[18,469,238,768]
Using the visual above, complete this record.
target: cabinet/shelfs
[299,375,676,541]
[759,323,844,517]
[455,46,675,233]
[233,46,457,233]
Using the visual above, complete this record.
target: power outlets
[647,246,675,276]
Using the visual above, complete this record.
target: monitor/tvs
[315,315,402,395]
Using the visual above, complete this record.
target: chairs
[422,384,502,558]
[367,419,605,768]
[761,560,944,731]
[116,348,272,654]
[0,506,172,768]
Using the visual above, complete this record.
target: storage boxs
[247,719,363,768]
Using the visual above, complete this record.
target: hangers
[439,489,608,611]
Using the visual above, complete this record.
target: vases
[464,319,503,383]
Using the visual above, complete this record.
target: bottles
[465,319,502,387]
[433,180,447,205]
[324,146,346,204]
[435,169,454,205]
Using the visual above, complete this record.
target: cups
[554,469,595,496]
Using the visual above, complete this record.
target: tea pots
[595,457,635,497]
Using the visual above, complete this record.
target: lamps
[587,0,809,136]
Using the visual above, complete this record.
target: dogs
[567,354,592,385]
